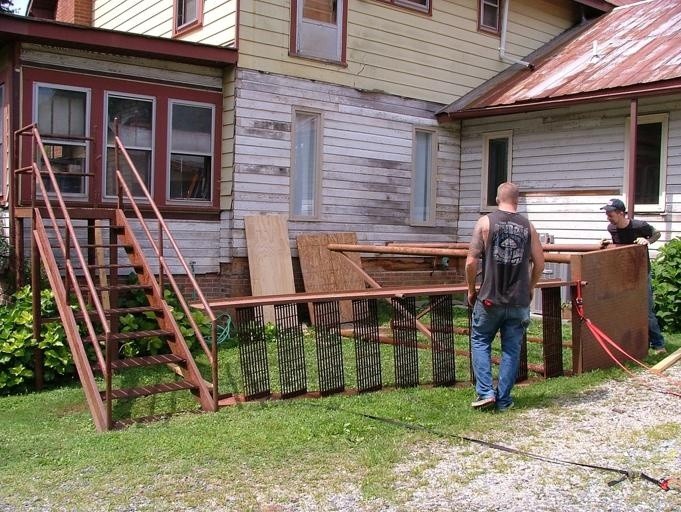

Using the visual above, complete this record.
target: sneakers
[654,344,669,356]
[471,394,497,409]
[498,398,517,413]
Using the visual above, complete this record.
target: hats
[600,198,626,212]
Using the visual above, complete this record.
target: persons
[601,198,667,356]
[466,181,545,415]
